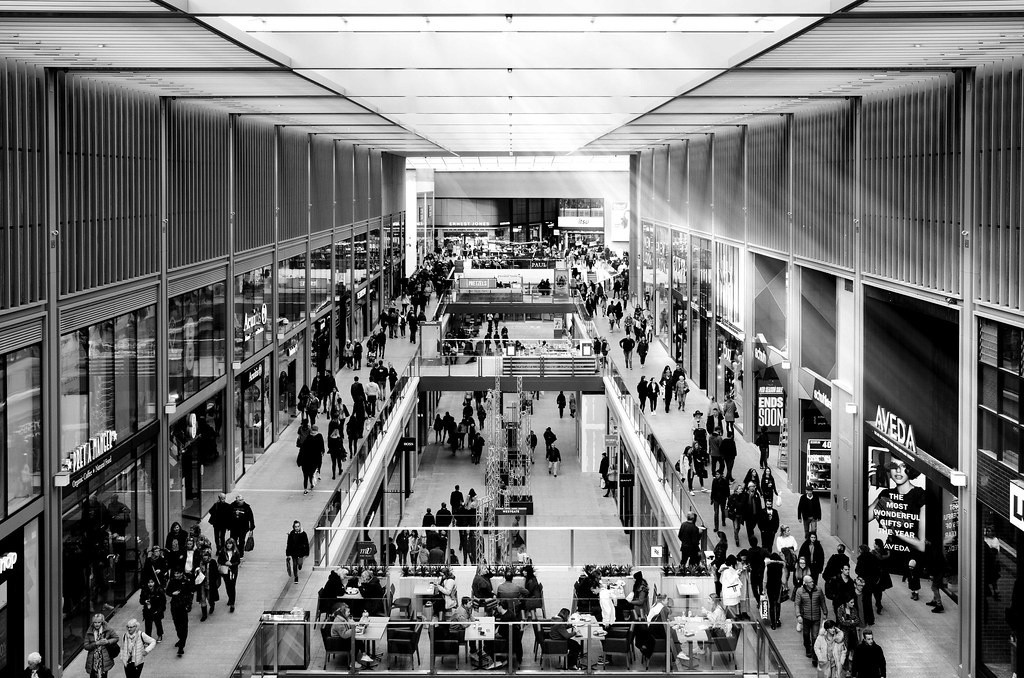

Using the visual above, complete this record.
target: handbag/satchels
[725,506,730,517]
[244,530,254,552]
[219,564,228,574]
[778,585,790,603]
[728,509,736,521]
[104,631,120,659]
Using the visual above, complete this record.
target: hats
[909,560,916,566]
[805,486,814,491]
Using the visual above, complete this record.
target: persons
[24,651,54,678]
[62,328,330,616]
[285,237,946,677]
[83,493,255,678]
[868,450,926,552]
[983,524,1002,601]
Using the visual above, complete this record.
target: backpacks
[825,576,838,600]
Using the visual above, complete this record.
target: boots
[200,605,208,622]
[910,592,919,600]
[208,598,215,614]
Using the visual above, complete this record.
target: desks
[599,584,625,599]
[358,617,391,662]
[399,577,442,617]
[343,576,390,605]
[467,617,495,661]
[660,573,717,615]
[569,616,599,659]
[573,627,605,671]
[336,579,363,619]
[676,583,700,617]
[490,576,526,620]
[465,624,495,671]
[673,616,704,661]
[601,571,638,597]
[414,584,435,620]
[674,630,708,672]
[355,628,390,671]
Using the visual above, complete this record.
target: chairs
[313,582,742,671]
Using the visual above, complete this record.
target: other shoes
[294,577,298,583]
[676,652,690,660]
[486,661,502,669]
[174,640,182,647]
[469,653,479,661]
[156,636,162,642]
[796,623,803,632]
[177,647,184,654]
[932,606,944,613]
[926,601,937,606]
[350,662,361,668]
[693,646,705,655]
[361,655,373,662]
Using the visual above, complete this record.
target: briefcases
[759,592,770,619]
